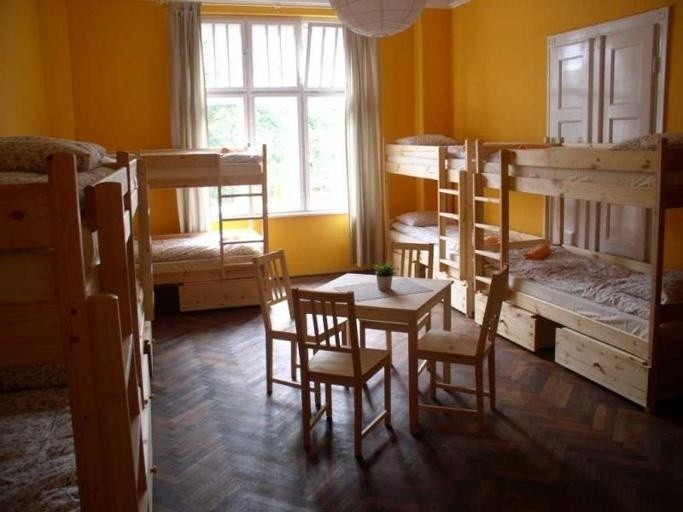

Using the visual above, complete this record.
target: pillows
[0,136,106,174]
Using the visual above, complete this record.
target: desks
[300,272,455,435]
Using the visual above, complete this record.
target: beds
[471,137,682,413]
[1,143,156,512]
[107,143,269,313]
[379,137,471,319]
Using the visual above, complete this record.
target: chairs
[251,249,350,406]
[290,287,393,459]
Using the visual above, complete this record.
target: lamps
[328,0,428,40]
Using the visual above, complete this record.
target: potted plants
[374,264,394,290]
